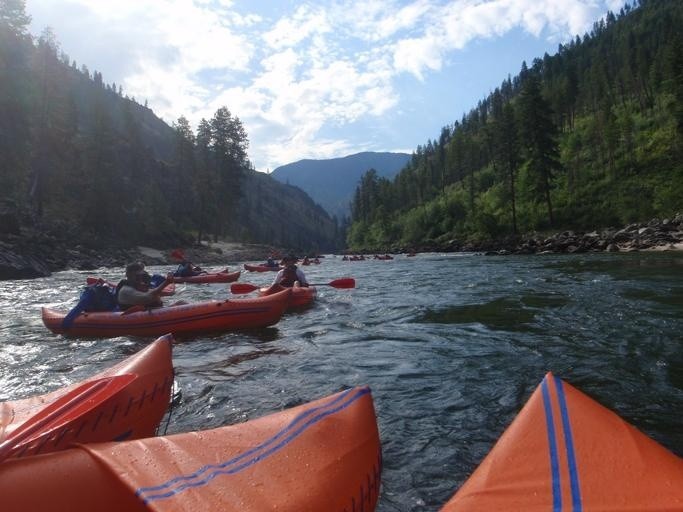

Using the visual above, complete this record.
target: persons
[137,271,189,308]
[261,255,319,268]
[117,262,176,310]
[271,254,308,287]
[178,260,207,277]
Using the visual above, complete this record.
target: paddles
[121,305,165,315]
[171,250,201,270]
[230,278,354,294]
[0,373,136,463]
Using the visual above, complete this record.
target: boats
[342,254,393,261]
[297,253,326,266]
[86,277,176,296]
[41,286,292,340]
[433,374,681,512]
[244,263,285,272]
[0,334,175,463]
[258,280,318,312]
[167,268,242,284]
[1,373,386,512]
